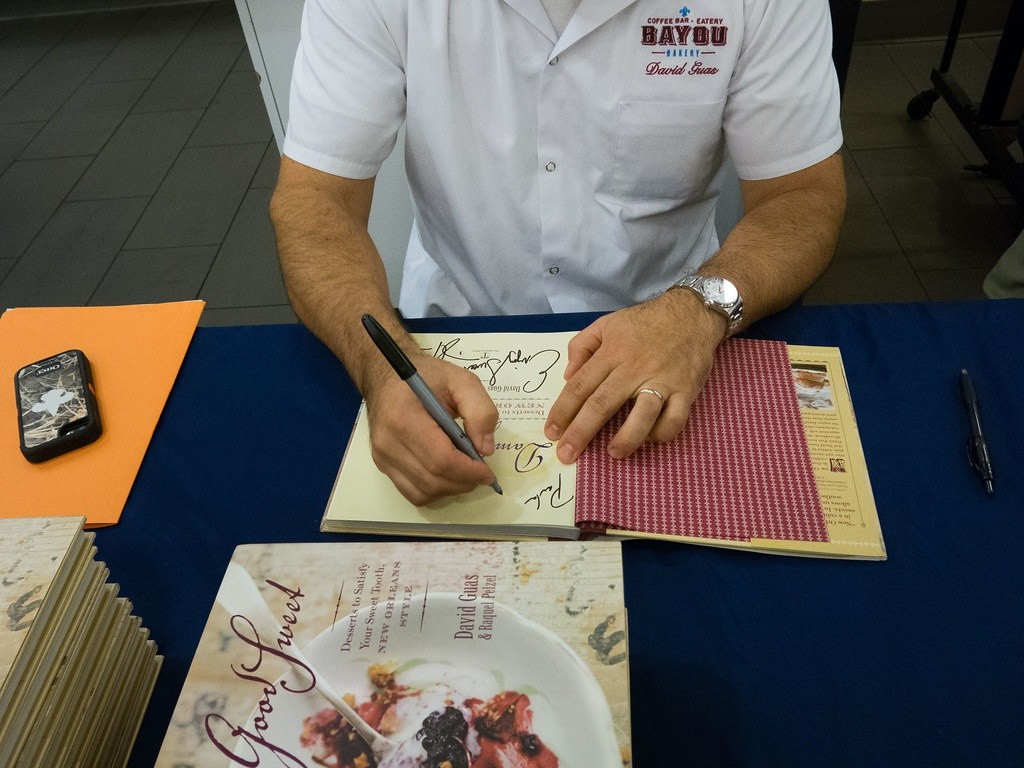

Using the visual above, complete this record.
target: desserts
[300,662,564,767]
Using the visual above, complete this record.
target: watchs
[665,274,746,343]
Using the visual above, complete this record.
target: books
[155,538,634,768]
[321,332,889,563]
[1,514,165,768]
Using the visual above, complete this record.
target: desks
[0,298,1024,767]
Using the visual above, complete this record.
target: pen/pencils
[959,367,994,496]
[362,312,506,497]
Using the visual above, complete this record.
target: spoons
[213,561,470,767]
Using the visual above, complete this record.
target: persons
[266,0,847,511]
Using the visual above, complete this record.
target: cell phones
[15,349,102,463]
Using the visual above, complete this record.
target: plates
[227,593,625,768]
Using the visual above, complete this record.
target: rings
[634,388,666,409]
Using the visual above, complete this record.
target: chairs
[238,3,744,316]
